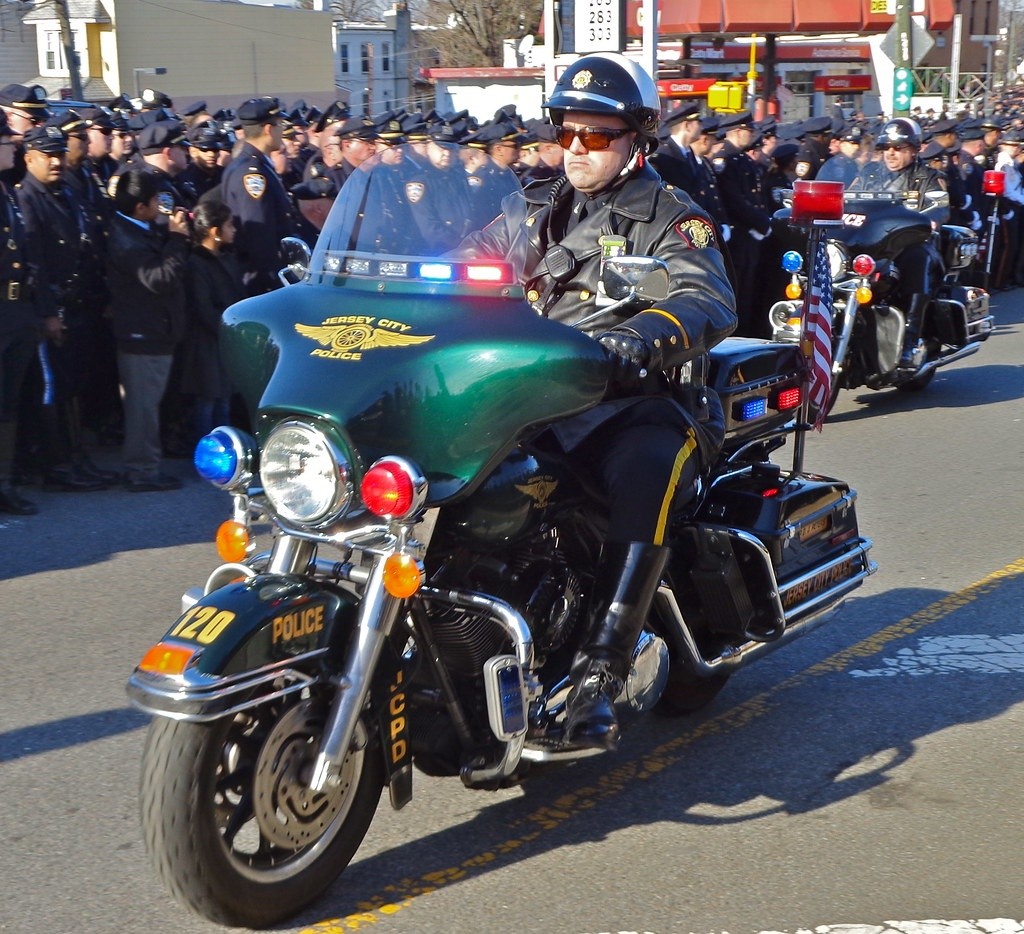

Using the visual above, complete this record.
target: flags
[802,229,833,434]
[995,151,1024,205]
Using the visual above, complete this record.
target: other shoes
[124,478,185,492]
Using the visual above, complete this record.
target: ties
[883,175,893,190]
[584,199,598,217]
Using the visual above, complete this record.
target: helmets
[874,118,923,152]
[540,54,662,138]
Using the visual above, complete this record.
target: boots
[0,398,121,515]
[563,539,673,750]
[896,293,932,372]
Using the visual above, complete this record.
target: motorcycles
[765,152,1007,427]
[117,139,881,931]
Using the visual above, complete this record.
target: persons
[641,102,886,340]
[107,169,193,492]
[909,83,1024,295]
[438,52,738,752]
[12,125,120,492]
[44,103,122,448]
[0,83,55,187]
[220,97,298,472]
[0,107,41,515]
[832,97,845,119]
[181,201,238,475]
[288,178,351,257]
[267,97,564,257]
[93,92,245,248]
[847,117,950,366]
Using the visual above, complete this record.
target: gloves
[595,331,649,394]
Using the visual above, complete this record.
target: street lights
[132,67,168,98]
[983,41,992,118]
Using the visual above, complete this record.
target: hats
[0,83,1024,156]
[236,95,293,125]
[21,126,71,155]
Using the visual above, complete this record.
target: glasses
[192,145,221,154]
[878,143,912,151]
[67,133,88,142]
[88,127,113,137]
[552,123,633,151]
[12,112,42,127]
